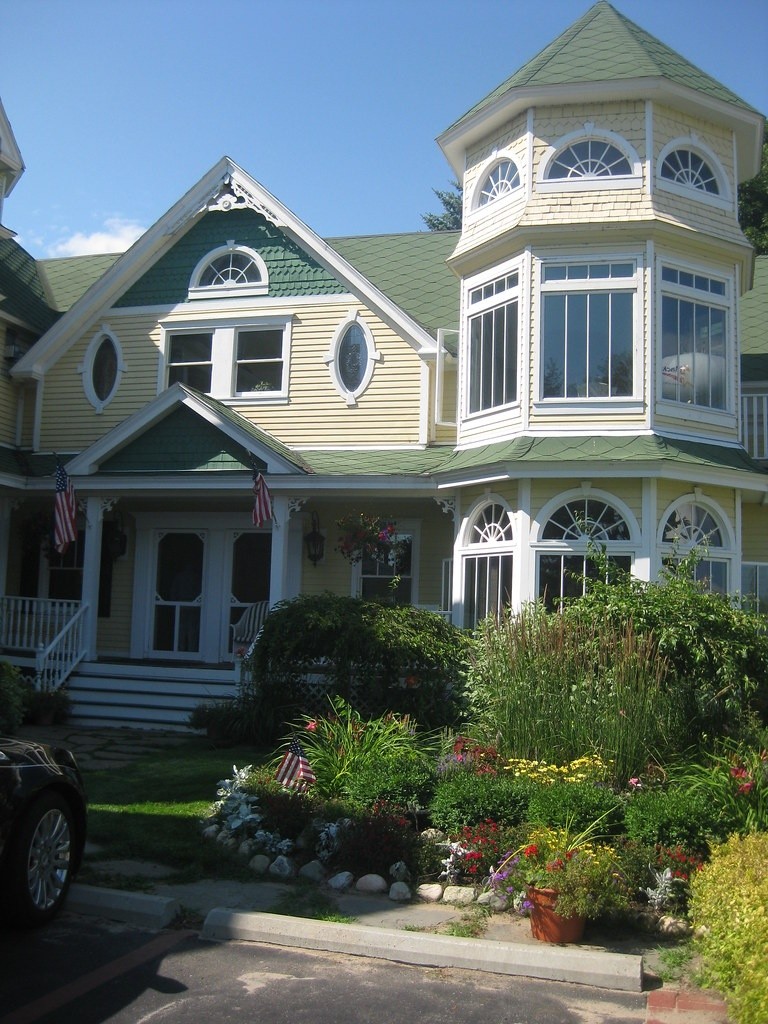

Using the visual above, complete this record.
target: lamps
[303,510,325,567]
[107,510,128,563]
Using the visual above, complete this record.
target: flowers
[487,803,636,919]
[332,496,409,569]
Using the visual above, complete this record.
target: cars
[0,732,91,926]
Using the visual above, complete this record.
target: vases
[523,881,588,943]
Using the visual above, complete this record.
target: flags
[254,474,273,527]
[54,459,75,552]
[276,737,316,794]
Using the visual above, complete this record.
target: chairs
[228,601,270,664]
